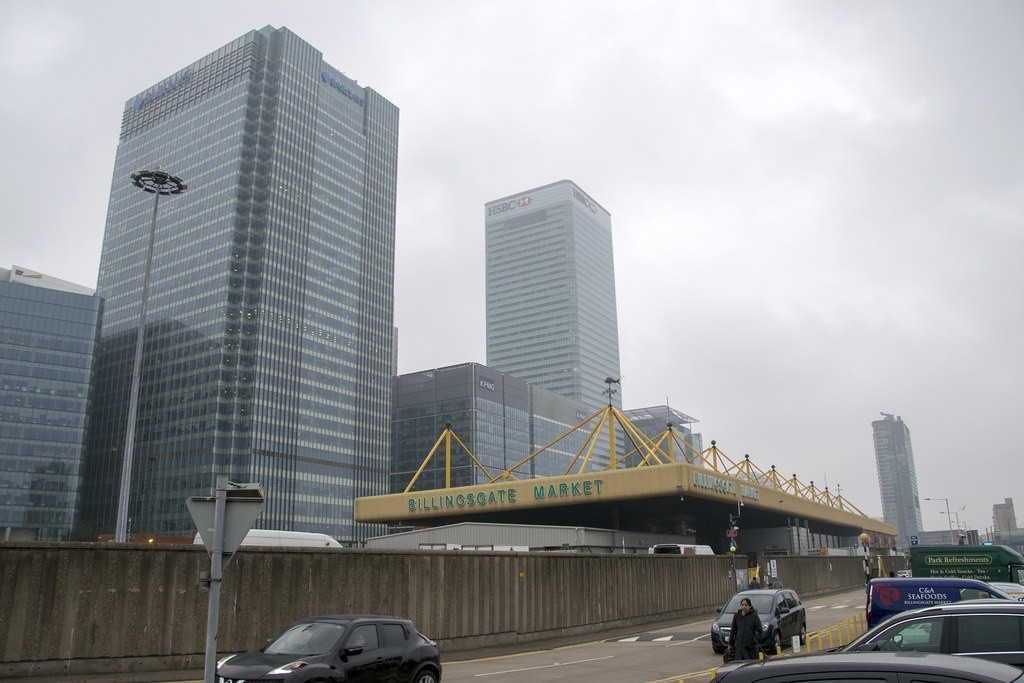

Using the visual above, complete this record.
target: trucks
[909,544,1024,585]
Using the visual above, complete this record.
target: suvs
[711,588,808,655]
[216,614,442,683]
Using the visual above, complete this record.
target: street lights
[951,520,971,530]
[940,512,960,535]
[115,163,189,542]
[924,498,954,545]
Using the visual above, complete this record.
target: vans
[189,526,345,548]
[867,578,1020,643]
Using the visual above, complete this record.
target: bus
[649,544,715,555]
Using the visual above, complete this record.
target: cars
[709,582,1024,683]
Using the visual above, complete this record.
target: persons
[769,577,782,589]
[751,577,760,590]
[728,597,762,660]
